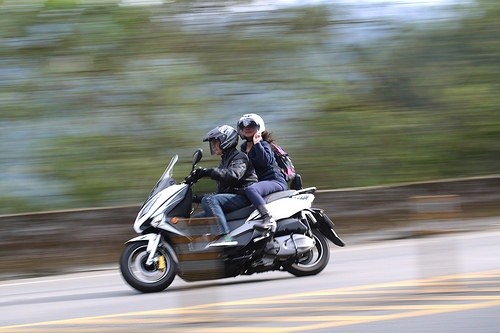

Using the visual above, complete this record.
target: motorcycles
[119,148,346,293]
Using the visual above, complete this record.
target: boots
[253,205,277,232]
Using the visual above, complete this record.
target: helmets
[202,124,239,152]
[237,113,265,138]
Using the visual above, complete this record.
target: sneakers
[209,234,238,249]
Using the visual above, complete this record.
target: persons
[192,125,258,246]
[236,113,288,233]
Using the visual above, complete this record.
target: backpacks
[268,138,296,181]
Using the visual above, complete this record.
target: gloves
[192,165,215,178]
